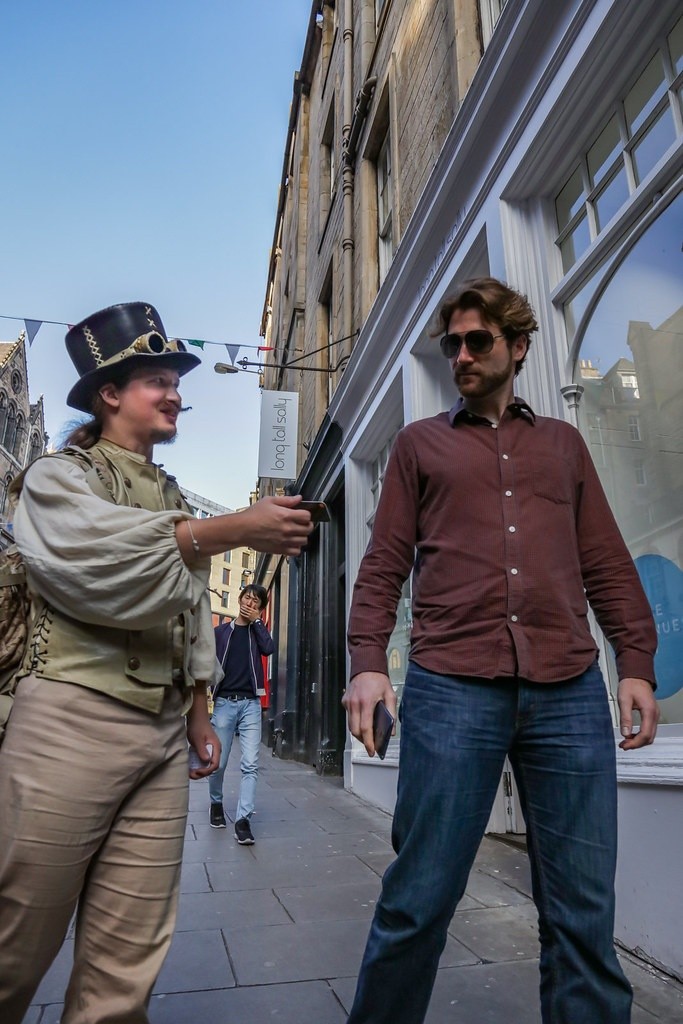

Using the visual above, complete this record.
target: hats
[66,302,202,414]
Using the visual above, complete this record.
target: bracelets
[186,519,200,559]
[253,618,261,624]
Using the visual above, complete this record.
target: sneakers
[235,818,255,844]
[209,803,226,828]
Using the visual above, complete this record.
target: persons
[0,302,315,1024]
[208,583,277,843]
[342,276,660,1024]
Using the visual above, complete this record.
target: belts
[224,695,251,702]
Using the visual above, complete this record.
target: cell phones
[371,700,395,761]
[292,500,331,523]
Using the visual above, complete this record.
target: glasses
[439,329,507,358]
[95,331,187,369]
